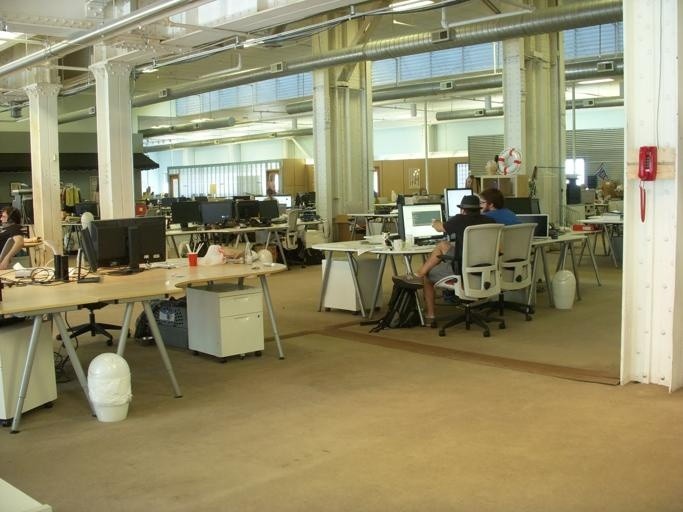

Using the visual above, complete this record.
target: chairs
[498,222,539,322]
[433,222,508,338]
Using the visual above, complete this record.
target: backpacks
[378,284,420,328]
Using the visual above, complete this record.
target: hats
[456,195,482,209]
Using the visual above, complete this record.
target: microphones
[0,219,13,224]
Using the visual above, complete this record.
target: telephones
[638,146,657,180]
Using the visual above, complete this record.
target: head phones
[7,208,18,224]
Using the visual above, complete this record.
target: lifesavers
[498,148,522,176]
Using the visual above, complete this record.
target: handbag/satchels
[250,216,272,226]
[215,216,235,227]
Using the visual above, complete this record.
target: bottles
[244,241,253,265]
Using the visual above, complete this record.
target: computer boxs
[503,197,540,214]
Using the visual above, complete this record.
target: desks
[576,216,622,268]
[0,257,288,434]
[165,218,328,271]
[312,235,589,322]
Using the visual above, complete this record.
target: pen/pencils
[185,243,204,254]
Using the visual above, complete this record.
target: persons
[442,187,522,306]
[0,207,24,269]
[392,197,497,331]
[263,189,278,204]
[95,184,99,215]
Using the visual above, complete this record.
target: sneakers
[421,314,435,326]
[391,273,423,289]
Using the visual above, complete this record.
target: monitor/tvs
[401,203,445,242]
[516,213,550,239]
[297,192,315,210]
[443,187,473,222]
[145,194,292,231]
[79,201,98,220]
[90,216,166,276]
[77,226,100,283]
[588,176,597,188]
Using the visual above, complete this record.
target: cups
[550,230,559,240]
[393,239,401,250]
[187,253,197,266]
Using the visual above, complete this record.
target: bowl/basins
[363,235,387,244]
